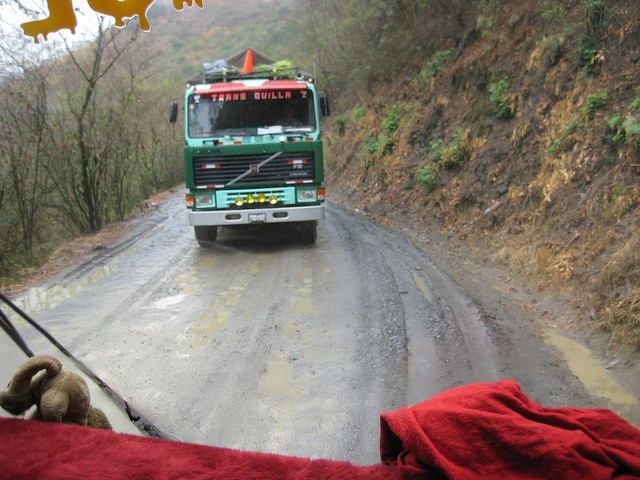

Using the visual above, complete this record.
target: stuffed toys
[0,355,112,431]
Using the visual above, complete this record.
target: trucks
[170,47,330,247]
